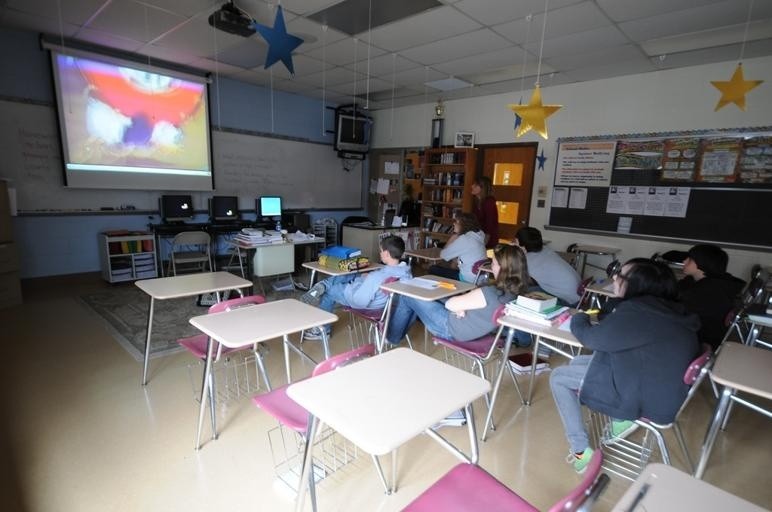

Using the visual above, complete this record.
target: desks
[611,462,769,512]
[481,308,598,443]
[694,342,772,479]
[302,256,384,290]
[226,236,325,297]
[188,297,339,450]
[286,346,493,512]
[404,246,445,266]
[134,271,254,387]
[378,275,477,349]
[584,281,617,309]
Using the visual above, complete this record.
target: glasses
[616,272,629,282]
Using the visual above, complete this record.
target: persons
[469,175,500,249]
[398,184,422,227]
[384,245,529,346]
[549,257,700,477]
[428,213,490,284]
[674,242,747,349]
[515,226,586,309]
[300,236,413,341]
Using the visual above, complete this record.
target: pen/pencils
[584,309,600,314]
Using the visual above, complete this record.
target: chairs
[744,278,764,303]
[167,230,213,276]
[586,357,714,481]
[252,343,392,512]
[575,276,596,309]
[574,244,621,279]
[344,277,413,353]
[471,258,492,284]
[706,307,750,345]
[430,302,526,430]
[177,295,273,439]
[400,447,609,512]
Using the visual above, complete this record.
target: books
[437,171,465,186]
[558,316,600,332]
[431,187,464,203]
[423,181,435,185]
[107,230,129,236]
[505,308,570,327]
[423,204,462,218]
[517,291,558,312]
[424,236,446,248]
[505,300,570,320]
[109,240,153,254]
[424,219,454,233]
[430,152,466,165]
[321,246,362,259]
[232,227,286,247]
[424,179,435,182]
[400,275,458,290]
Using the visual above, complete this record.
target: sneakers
[564,447,593,476]
[303,330,330,340]
[601,419,638,446]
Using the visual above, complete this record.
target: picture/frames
[454,131,475,149]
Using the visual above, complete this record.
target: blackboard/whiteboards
[0,96,364,213]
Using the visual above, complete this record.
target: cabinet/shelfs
[314,218,338,261]
[368,148,424,223]
[421,149,478,242]
[99,231,158,283]
[342,223,420,261]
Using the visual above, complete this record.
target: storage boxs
[378,154,401,178]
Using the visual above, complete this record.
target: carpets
[77,290,344,362]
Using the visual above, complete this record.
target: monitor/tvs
[212,195,238,220]
[260,195,282,217]
[161,194,193,221]
[335,114,372,152]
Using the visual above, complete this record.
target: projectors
[208,1,256,38]
